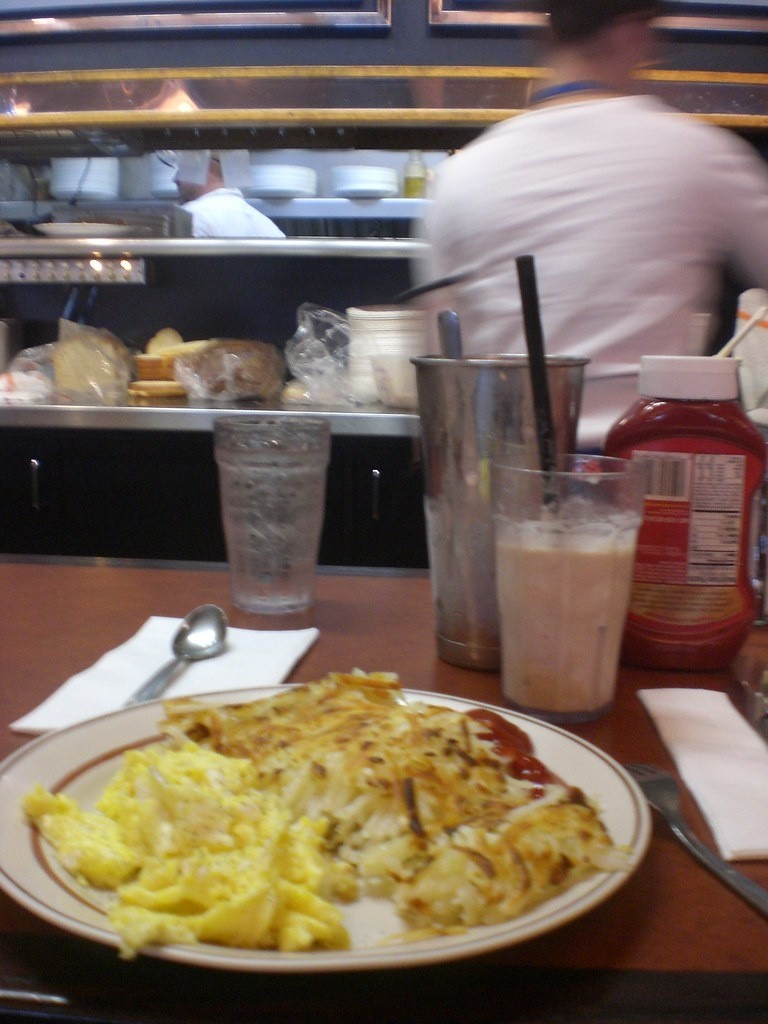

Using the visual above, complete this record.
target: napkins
[636,689,768,862]
[8,615,321,737]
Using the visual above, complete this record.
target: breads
[51,333,135,404]
[128,327,280,397]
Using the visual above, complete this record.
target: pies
[169,672,621,932]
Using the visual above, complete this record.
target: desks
[0,552,768,973]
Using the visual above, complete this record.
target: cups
[489,449,644,722]
[410,351,589,673]
[209,417,333,615]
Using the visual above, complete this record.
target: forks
[617,757,768,933]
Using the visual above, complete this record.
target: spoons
[119,604,228,721]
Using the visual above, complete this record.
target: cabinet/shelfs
[0,121,429,438]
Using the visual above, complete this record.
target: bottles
[402,149,428,199]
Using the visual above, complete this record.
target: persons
[411,0,768,451]
[173,150,286,237]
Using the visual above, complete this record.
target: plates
[48,157,120,199]
[0,677,649,972]
[331,165,400,199]
[346,304,430,408]
[150,152,182,199]
[33,221,134,238]
[244,164,318,198]
